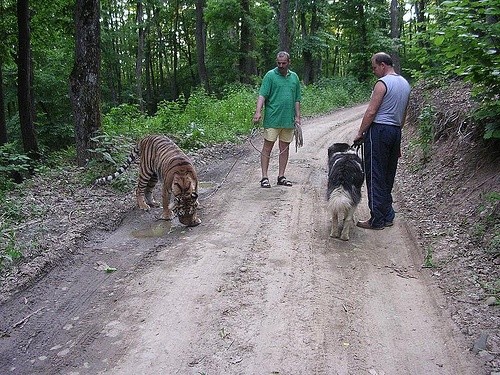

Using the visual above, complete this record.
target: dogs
[324,141,366,243]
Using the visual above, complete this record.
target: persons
[251,51,302,187]
[352,51,410,229]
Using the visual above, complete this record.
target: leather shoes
[385,220,393,227]
[357,219,384,230]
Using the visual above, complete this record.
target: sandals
[259,177,271,188]
[278,175,291,186]
